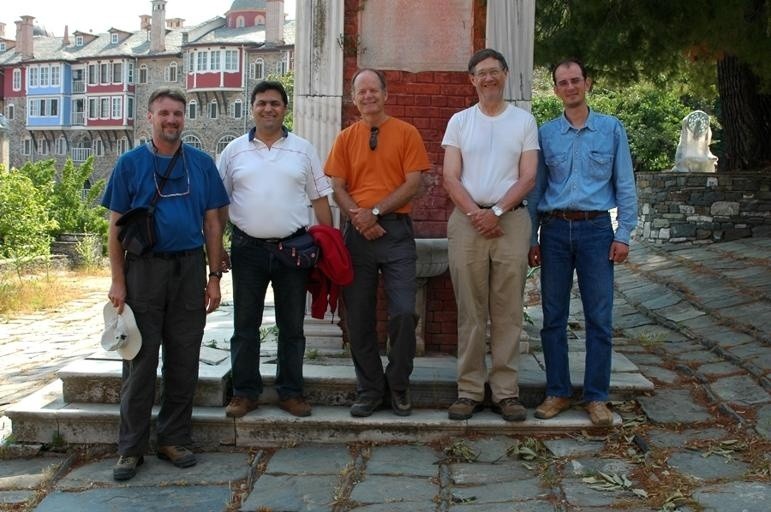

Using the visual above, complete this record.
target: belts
[143,247,202,259]
[233,225,305,243]
[379,213,408,220]
[547,210,600,220]
[478,200,527,210]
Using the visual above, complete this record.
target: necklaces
[144,144,194,202]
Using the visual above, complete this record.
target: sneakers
[158,445,197,467]
[279,395,312,416]
[388,383,413,415]
[535,397,571,418]
[490,398,527,421]
[586,401,614,425]
[449,399,486,419]
[226,396,259,417]
[113,455,144,480]
[351,397,384,415]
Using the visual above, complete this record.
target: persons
[216,81,336,418]
[441,49,541,423]
[527,58,638,427]
[98,84,232,481]
[323,68,431,415]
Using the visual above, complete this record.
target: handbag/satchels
[271,235,323,272]
[115,206,156,255]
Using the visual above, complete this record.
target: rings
[356,225,360,231]
[478,226,482,230]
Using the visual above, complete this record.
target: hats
[101,302,143,360]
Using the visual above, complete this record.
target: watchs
[373,208,383,217]
[492,206,504,217]
[208,269,223,281]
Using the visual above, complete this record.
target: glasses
[154,169,190,197]
[370,127,378,150]
